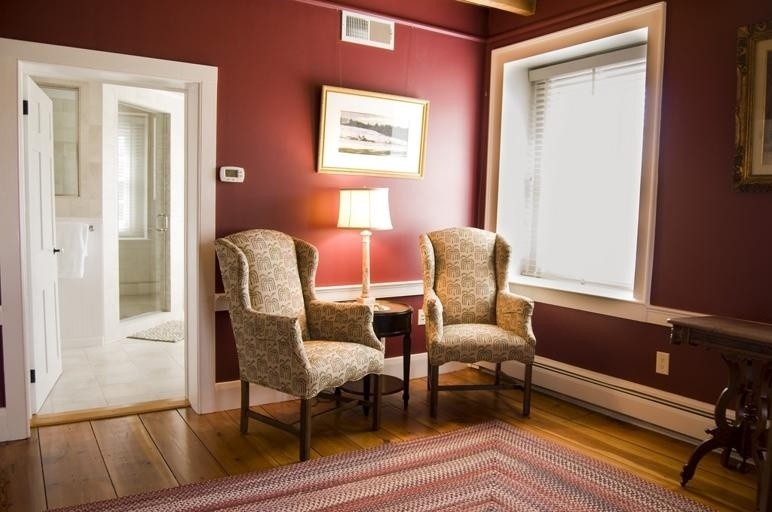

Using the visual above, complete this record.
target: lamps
[336,187,393,312]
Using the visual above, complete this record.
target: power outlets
[656,351,669,375]
[418,310,426,326]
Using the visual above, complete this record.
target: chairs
[418,227,536,419]
[213,229,385,463]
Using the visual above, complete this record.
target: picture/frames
[731,21,772,193]
[316,84,429,181]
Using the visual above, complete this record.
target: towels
[56,221,88,279]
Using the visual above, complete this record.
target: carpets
[38,418,719,512]
[127,320,184,343]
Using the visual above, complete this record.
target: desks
[335,300,414,417]
[666,315,772,512]
